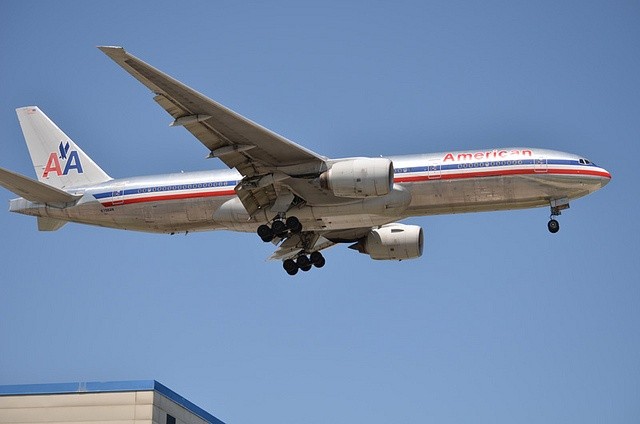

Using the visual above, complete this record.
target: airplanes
[0,45,610,275]
